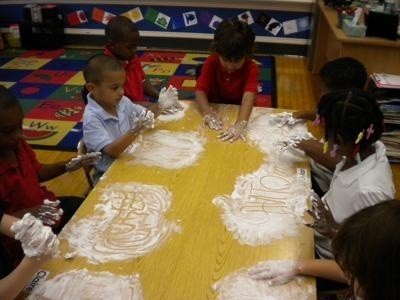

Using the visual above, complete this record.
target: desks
[312,0,400,91]
[14,99,317,300]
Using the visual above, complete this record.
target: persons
[0,84,103,255]
[102,15,184,117]
[80,53,180,183]
[249,198,400,300]
[304,86,400,290]
[269,56,369,196]
[0,210,61,299]
[194,18,261,144]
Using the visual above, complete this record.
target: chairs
[77,136,103,190]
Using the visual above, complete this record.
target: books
[369,72,400,164]
